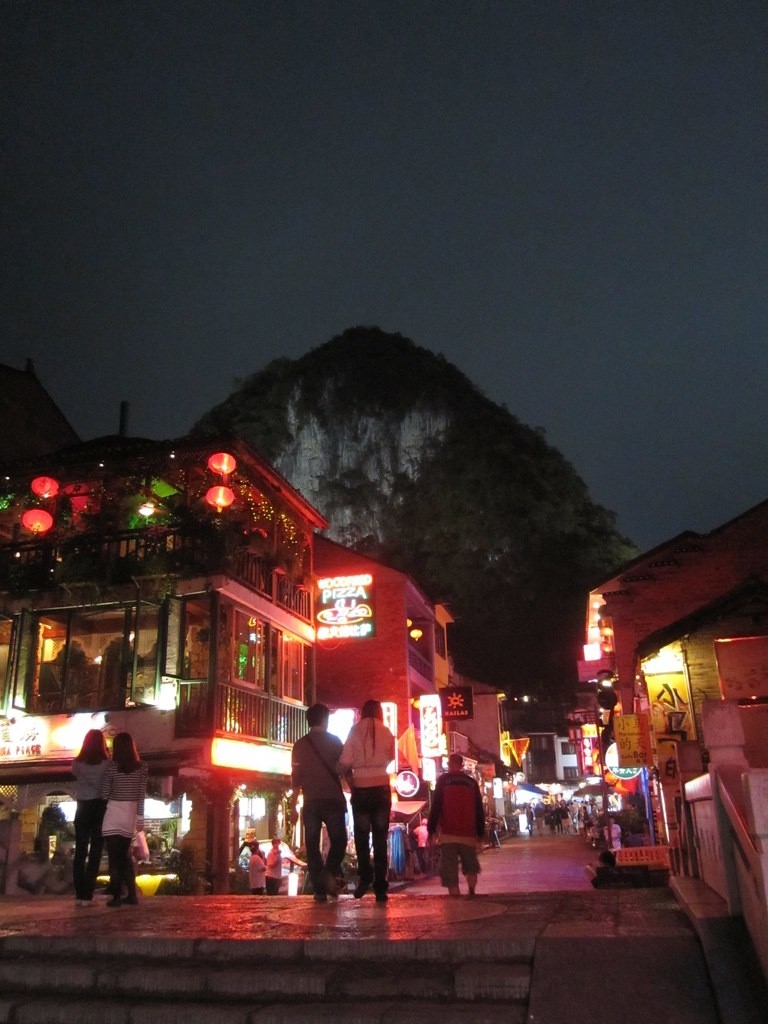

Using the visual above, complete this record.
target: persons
[511,797,637,850]
[72,729,146,905]
[427,755,485,896]
[290,703,352,902]
[336,701,395,902]
[238,836,283,895]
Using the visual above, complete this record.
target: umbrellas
[574,785,615,798]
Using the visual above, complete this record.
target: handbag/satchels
[128,829,150,864]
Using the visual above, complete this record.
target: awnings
[523,785,550,796]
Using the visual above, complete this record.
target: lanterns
[204,452,237,513]
[21,477,59,536]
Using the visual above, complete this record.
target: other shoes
[376,890,389,902]
[75,898,92,907]
[121,895,138,904]
[354,875,372,898]
[325,868,339,898]
[314,889,327,901]
[105,897,122,907]
